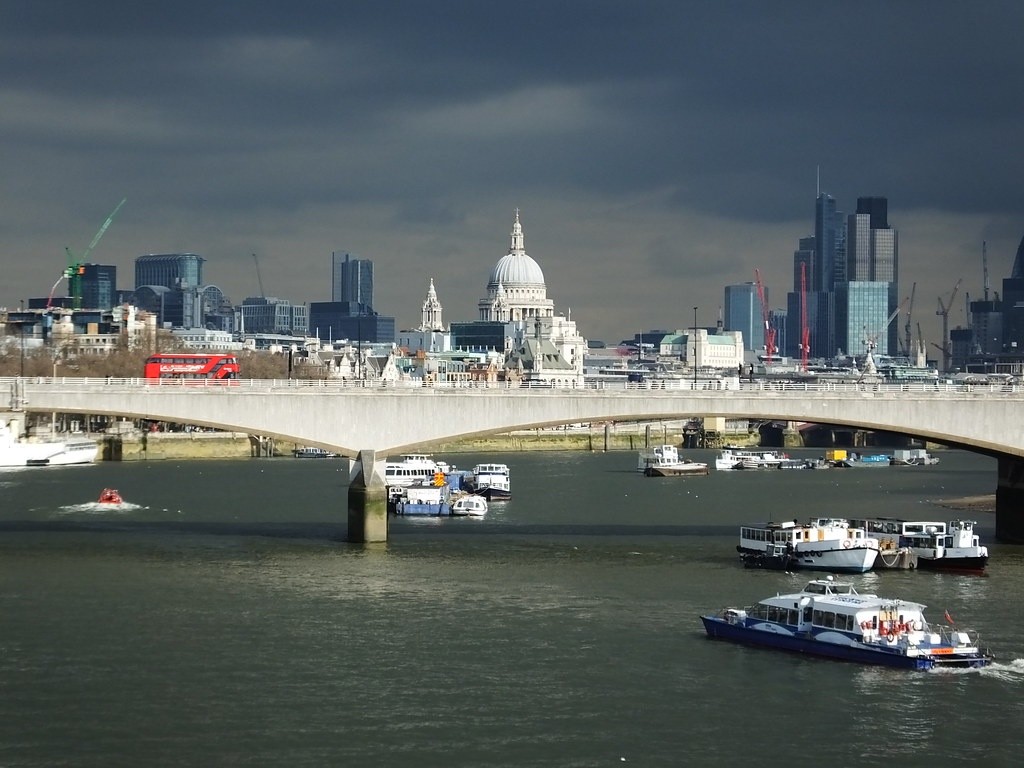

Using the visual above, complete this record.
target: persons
[382,378,387,387]
[507,377,511,388]
[422,375,433,387]
[933,378,940,392]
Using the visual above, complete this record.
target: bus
[143,352,242,388]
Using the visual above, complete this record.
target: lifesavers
[886,632,895,642]
[843,540,852,548]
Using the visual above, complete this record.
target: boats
[472,462,512,501]
[698,574,996,673]
[637,425,685,472]
[643,464,710,477]
[96,487,122,504]
[735,515,989,573]
[453,493,488,516]
[0,430,99,464]
[714,447,940,472]
[385,452,450,485]
[292,445,335,457]
